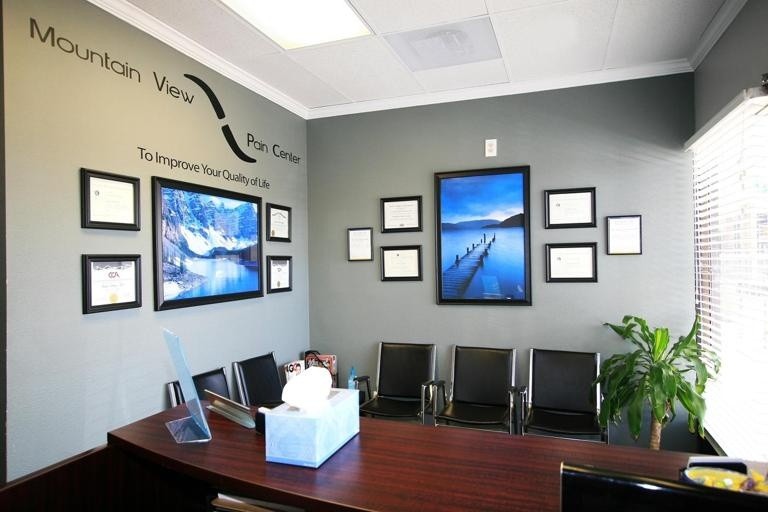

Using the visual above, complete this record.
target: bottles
[347,368,358,389]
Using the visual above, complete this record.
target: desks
[106,399,768,512]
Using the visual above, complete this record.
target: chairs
[519,348,609,443]
[432,345,518,435]
[232,350,283,406]
[353,341,437,425]
[192,367,230,399]
[558,461,768,512]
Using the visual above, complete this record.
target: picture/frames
[81,253,142,314]
[347,227,374,261]
[80,168,141,231]
[545,241,597,283]
[266,254,293,294]
[434,165,532,307]
[151,176,264,311]
[544,186,596,229]
[379,245,423,281]
[265,202,292,242]
[380,195,423,233]
[607,215,642,254]
